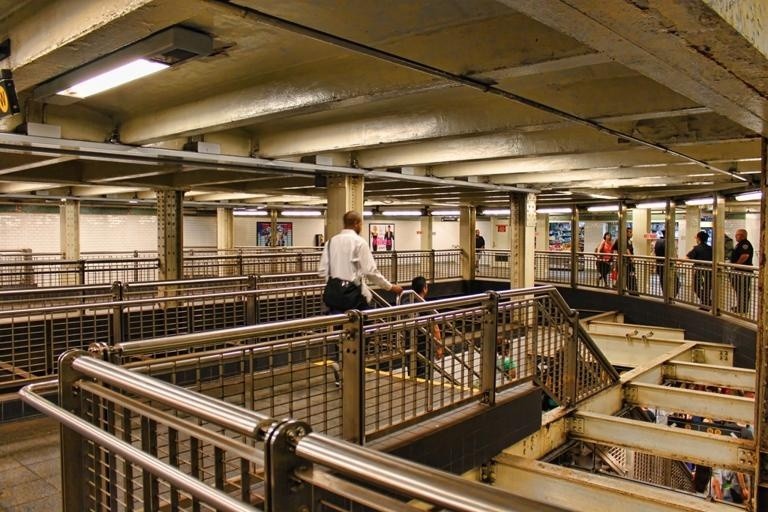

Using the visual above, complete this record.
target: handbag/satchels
[322,277,363,314]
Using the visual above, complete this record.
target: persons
[371,225,378,251]
[676,230,712,306]
[741,425,754,439]
[728,228,755,314]
[476,229,486,272]
[729,472,749,504]
[707,386,718,393]
[317,210,405,387]
[691,465,722,500]
[493,341,517,387]
[614,227,639,296]
[383,225,394,250]
[656,229,681,297]
[400,276,434,381]
[593,226,614,288]
